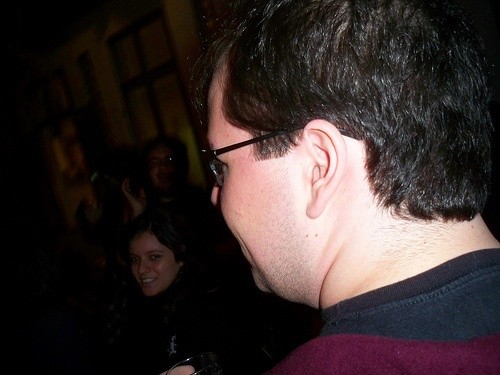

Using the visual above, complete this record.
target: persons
[124,210,232,375]
[197,0,500,375]
[121,135,235,321]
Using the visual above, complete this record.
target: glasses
[201,122,308,188]
